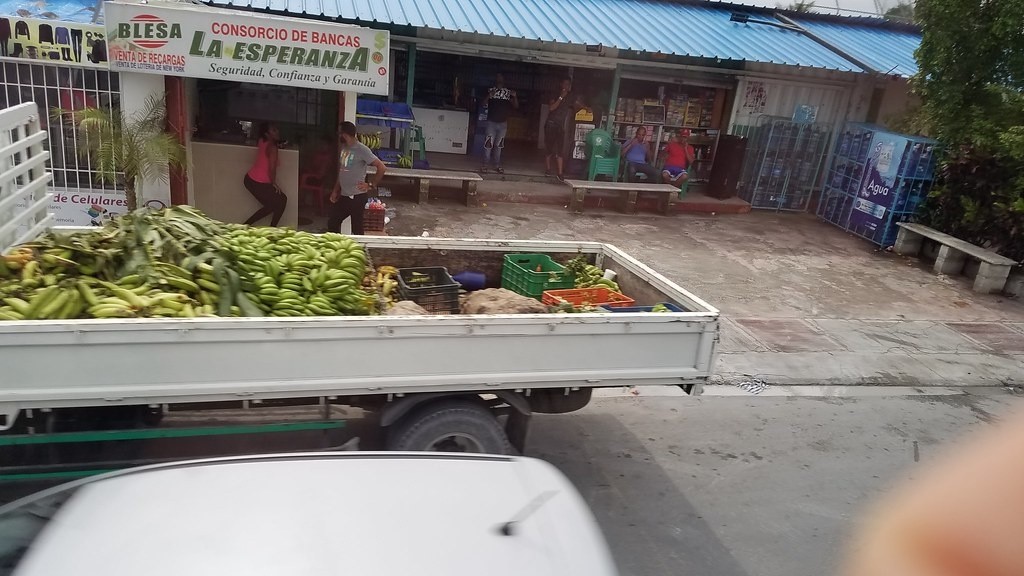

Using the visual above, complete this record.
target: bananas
[0,223,431,321]
[398,155,413,167]
[358,133,381,149]
[573,264,621,294]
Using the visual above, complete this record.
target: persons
[243,121,287,228]
[328,121,386,235]
[544,76,587,182]
[662,128,695,187]
[480,71,519,175]
[621,126,660,183]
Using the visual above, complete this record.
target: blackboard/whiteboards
[642,105,666,123]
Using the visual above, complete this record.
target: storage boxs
[395,266,462,314]
[363,208,385,231]
[376,147,405,167]
[542,287,635,308]
[501,253,575,301]
[600,302,686,312]
[357,99,415,129]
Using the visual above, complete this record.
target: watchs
[368,182,374,188]
[559,96,563,101]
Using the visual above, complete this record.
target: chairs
[299,160,328,208]
[657,149,698,201]
[585,128,621,181]
[563,179,682,214]
[622,159,646,183]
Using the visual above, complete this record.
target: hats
[680,129,690,138]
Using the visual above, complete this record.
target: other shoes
[480,166,488,173]
[545,171,551,176]
[556,175,565,182]
[494,167,504,173]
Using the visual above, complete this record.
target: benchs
[365,166,483,208]
[894,222,1018,294]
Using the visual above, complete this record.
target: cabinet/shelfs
[598,117,730,182]
[737,114,830,214]
[816,123,945,251]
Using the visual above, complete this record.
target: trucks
[0,102,719,456]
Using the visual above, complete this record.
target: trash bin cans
[707,135,750,198]
[51,125,82,183]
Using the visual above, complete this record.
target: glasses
[336,131,345,137]
